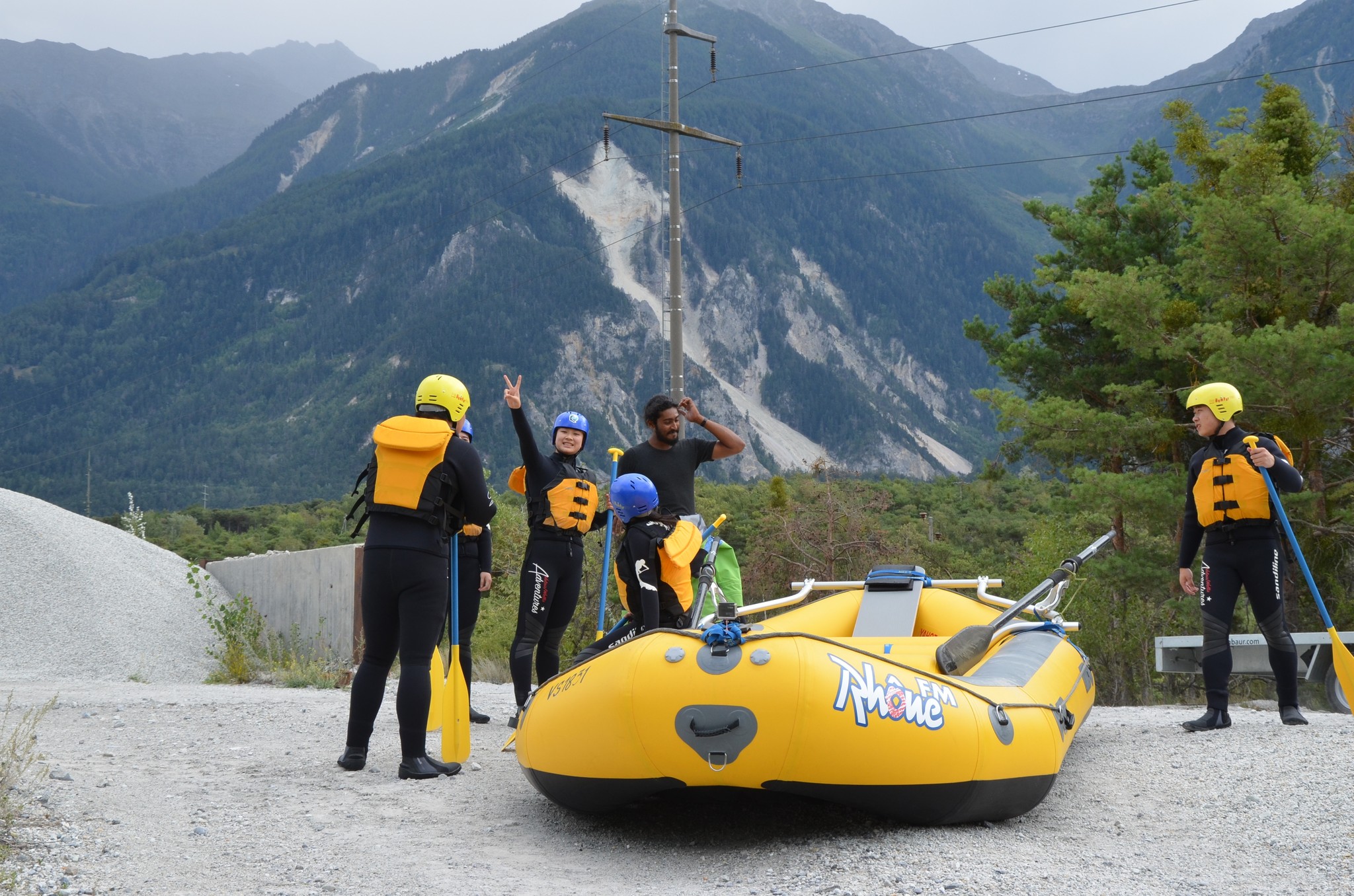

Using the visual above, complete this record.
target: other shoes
[398,755,461,780]
[469,706,491,723]
[1279,706,1309,725]
[337,746,368,771]
[1181,708,1231,731]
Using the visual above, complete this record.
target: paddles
[425,645,444,732]
[595,448,624,642]
[501,513,726,752]
[442,533,471,764]
[935,530,1117,677]
[1242,435,1354,716]
[686,536,720,630]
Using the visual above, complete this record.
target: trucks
[1155,632,1354,715]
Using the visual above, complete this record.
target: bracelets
[701,417,707,427]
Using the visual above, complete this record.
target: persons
[1177,383,1305,732]
[336,374,498,781]
[466,374,748,725]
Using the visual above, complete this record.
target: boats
[502,565,1097,834]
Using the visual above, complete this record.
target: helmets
[462,419,474,444]
[551,411,590,451]
[415,374,471,422]
[1186,382,1243,421]
[609,473,659,523]
